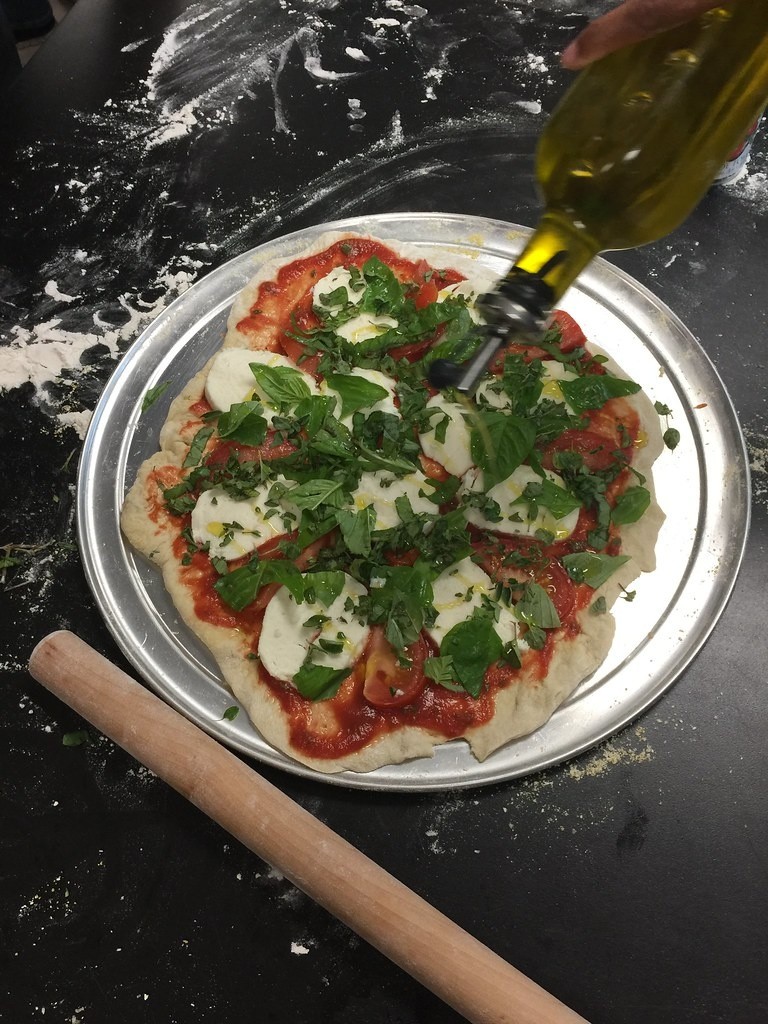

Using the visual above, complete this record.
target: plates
[75,213,751,789]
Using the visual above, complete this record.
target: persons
[557,0,725,69]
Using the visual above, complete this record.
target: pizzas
[116,232,665,773]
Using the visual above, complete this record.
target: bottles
[453,0,768,400]
